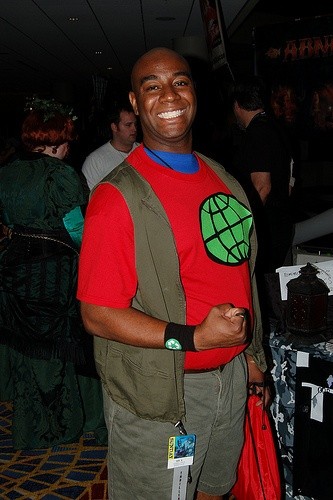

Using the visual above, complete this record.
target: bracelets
[249,382,269,387]
[163,323,199,352]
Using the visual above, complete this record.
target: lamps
[286,262,330,337]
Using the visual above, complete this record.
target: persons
[222,73,298,319]
[81,102,142,193]
[0,97,107,449]
[75,46,270,500]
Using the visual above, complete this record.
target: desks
[262,326,333,500]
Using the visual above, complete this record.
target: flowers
[24,96,78,123]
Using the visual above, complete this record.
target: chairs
[0,231,109,450]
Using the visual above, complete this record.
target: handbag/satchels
[231,394,282,500]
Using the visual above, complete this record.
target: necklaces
[142,140,172,169]
